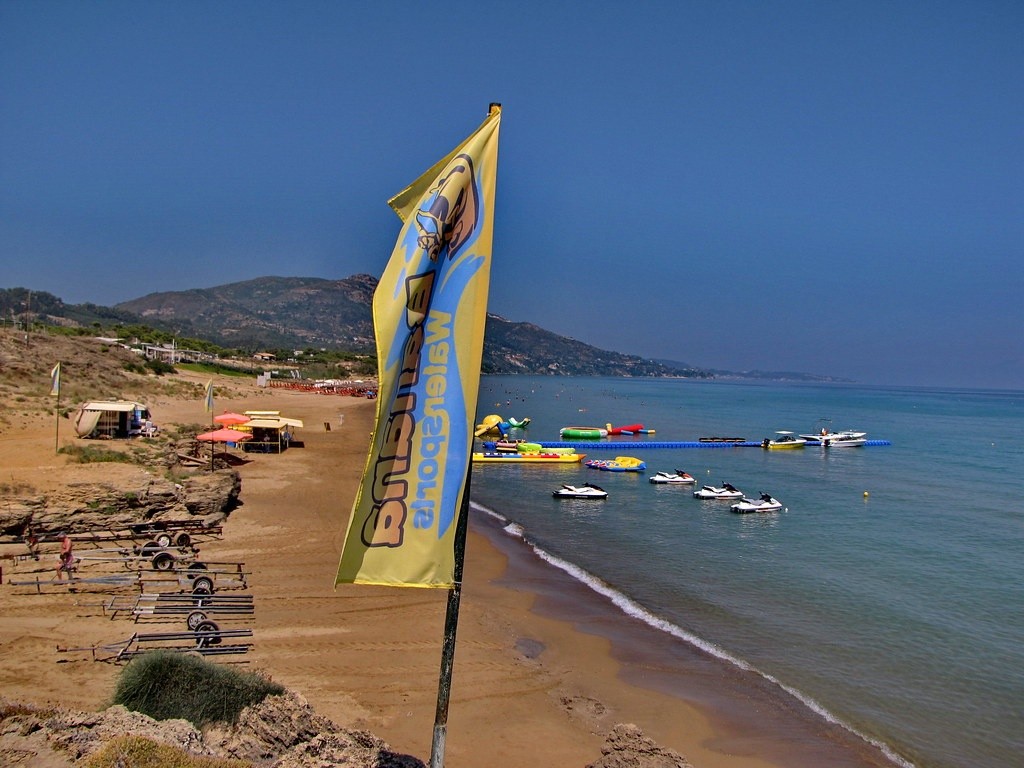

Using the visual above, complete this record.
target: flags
[206,379,213,412]
[49,361,60,396]
[333,107,502,588]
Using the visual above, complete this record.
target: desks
[128,428,156,437]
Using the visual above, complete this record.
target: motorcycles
[730,492,783,514]
[649,468,695,484]
[692,480,743,500]
[553,483,608,500]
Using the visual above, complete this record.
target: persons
[489,382,644,406]
[264,433,272,453]
[56,531,73,580]
[24,520,39,559]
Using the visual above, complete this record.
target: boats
[472,452,587,463]
[820,437,867,447]
[799,430,867,441]
[586,456,647,471]
[761,436,807,449]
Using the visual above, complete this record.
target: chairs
[269,381,378,398]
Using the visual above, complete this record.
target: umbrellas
[197,410,253,458]
